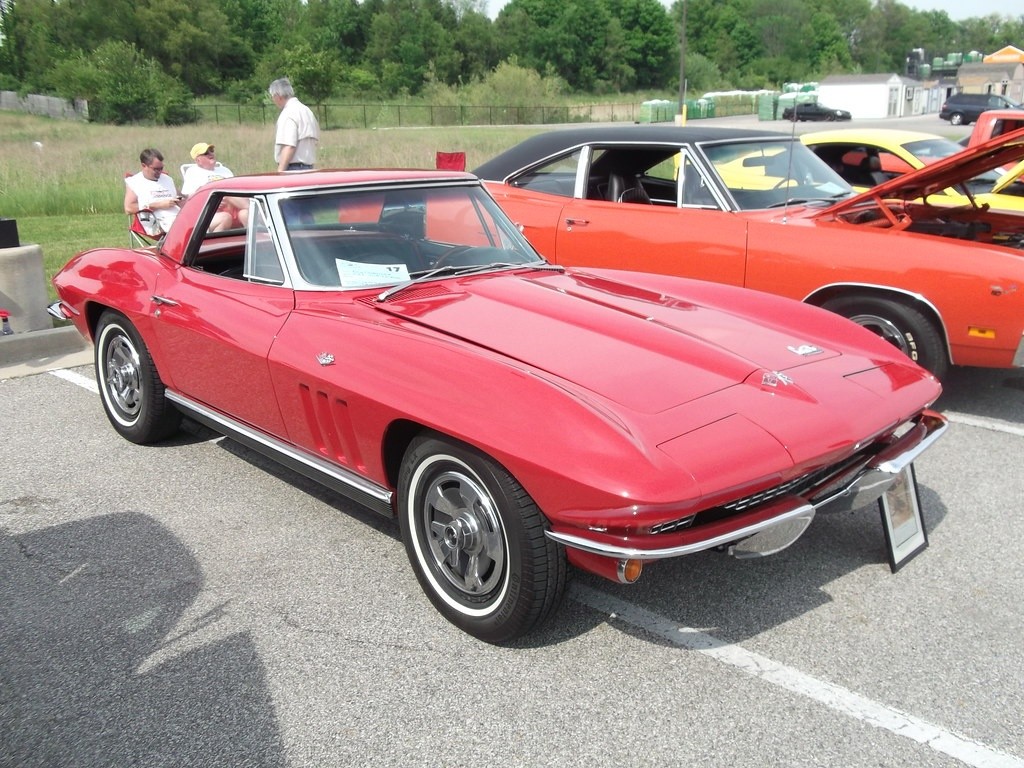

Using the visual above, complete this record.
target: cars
[939,93,1024,125]
[783,102,851,122]
[43,168,948,645]
[340,127,1024,383]
[705,129,1024,212]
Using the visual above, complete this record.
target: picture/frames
[881,460,929,575]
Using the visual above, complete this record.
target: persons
[124,149,233,236]
[181,143,251,228]
[269,78,321,224]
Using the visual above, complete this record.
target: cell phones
[177,197,182,200]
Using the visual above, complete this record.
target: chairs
[861,155,890,186]
[125,171,169,248]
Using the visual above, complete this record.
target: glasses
[147,167,163,171]
[204,149,213,154]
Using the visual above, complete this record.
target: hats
[190,142,214,160]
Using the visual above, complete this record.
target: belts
[288,163,311,167]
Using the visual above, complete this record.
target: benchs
[218,236,424,289]
[525,174,648,206]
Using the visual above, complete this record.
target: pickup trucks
[841,110,1024,184]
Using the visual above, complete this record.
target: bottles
[0,316,14,336]
